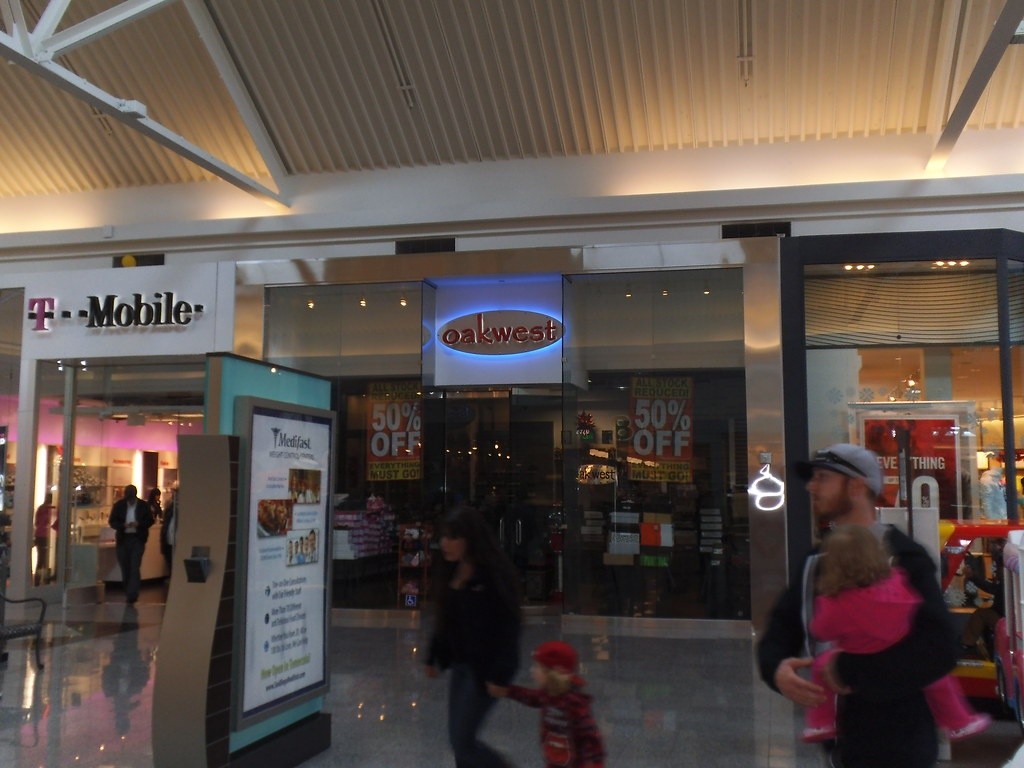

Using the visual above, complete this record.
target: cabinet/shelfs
[396,521,436,609]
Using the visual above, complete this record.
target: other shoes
[947,714,991,739]
[797,724,835,743]
[125,594,139,604]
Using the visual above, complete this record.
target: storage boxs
[602,511,675,568]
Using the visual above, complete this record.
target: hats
[531,640,587,688]
[123,485,137,503]
[809,443,883,507]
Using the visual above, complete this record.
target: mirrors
[57,464,132,509]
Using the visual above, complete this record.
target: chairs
[0,590,47,670]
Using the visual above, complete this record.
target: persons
[290,470,320,505]
[424,499,520,767]
[257,500,292,537]
[160,502,172,593]
[799,522,991,742]
[146,488,164,517]
[486,642,605,768]
[108,485,152,607]
[31,493,52,575]
[100,603,151,737]
[288,529,319,564]
[757,443,961,768]
